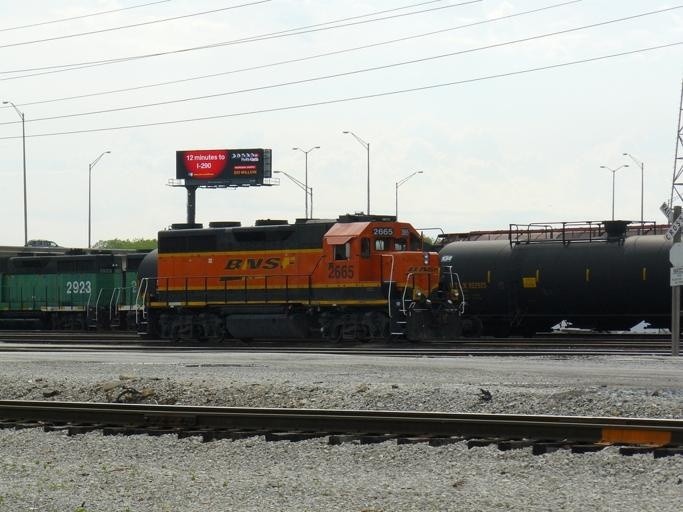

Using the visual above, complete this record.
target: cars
[28,239,65,248]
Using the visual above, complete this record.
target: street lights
[599,153,644,222]
[88,150,113,250]
[273,145,321,219]
[342,130,370,218]
[394,169,423,221]
[1,101,28,247]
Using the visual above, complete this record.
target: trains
[0,213,682,343]
